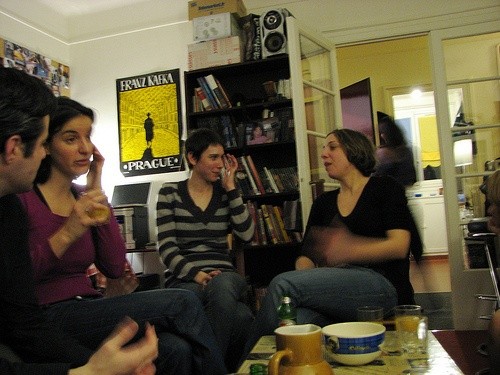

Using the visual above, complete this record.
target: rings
[85,209,89,213]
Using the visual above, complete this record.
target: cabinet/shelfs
[408,197,448,257]
[185,54,302,249]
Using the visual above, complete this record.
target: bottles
[250,363,267,375]
[276,296,297,328]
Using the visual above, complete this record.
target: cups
[395,305,422,344]
[268,324,333,375]
[398,316,429,371]
[357,306,384,325]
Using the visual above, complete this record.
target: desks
[238,330,500,375]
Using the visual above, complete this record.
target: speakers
[259,9,288,59]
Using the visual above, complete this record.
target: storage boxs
[187,0,246,71]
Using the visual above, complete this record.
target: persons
[0,67,158,375]
[370,111,416,186]
[156,127,255,366]
[15,95,228,375]
[244,129,415,365]
[246,124,276,144]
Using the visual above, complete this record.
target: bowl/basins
[322,322,386,365]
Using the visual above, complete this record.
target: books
[264,107,293,143]
[218,155,299,195]
[192,75,231,112]
[275,78,294,99]
[242,199,303,245]
[186,115,253,148]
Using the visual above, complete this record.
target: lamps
[454,139,473,194]
